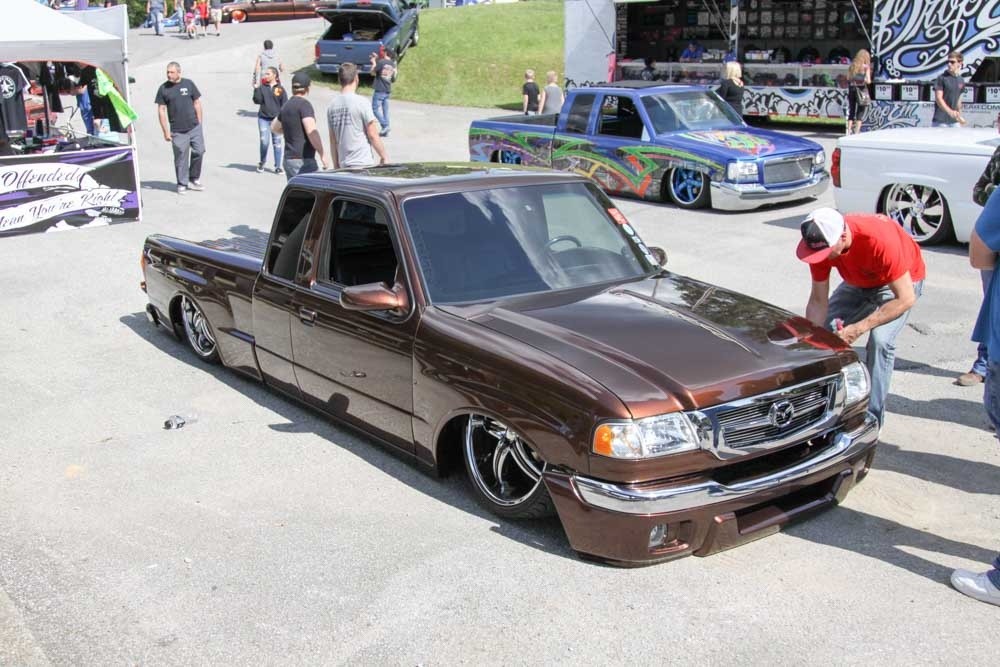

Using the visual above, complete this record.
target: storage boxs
[867,81,1000,104]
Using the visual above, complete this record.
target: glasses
[946,61,959,64]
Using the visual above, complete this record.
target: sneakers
[951,568,1000,606]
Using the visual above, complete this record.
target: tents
[0,0,142,220]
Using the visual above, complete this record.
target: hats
[796,207,844,263]
[292,72,310,88]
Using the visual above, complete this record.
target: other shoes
[275,168,285,175]
[958,371,983,385]
[257,162,264,172]
[187,180,204,190]
[178,184,187,194]
[380,127,390,136]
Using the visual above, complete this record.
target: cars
[162,12,181,27]
[207,0,322,23]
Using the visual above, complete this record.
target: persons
[251,39,400,221]
[718,61,744,118]
[639,56,662,82]
[931,52,967,128]
[846,49,871,135]
[538,70,564,116]
[958,111,1000,385]
[155,61,206,195]
[35,0,222,135]
[796,207,926,431]
[522,69,540,116]
[948,185,1000,607]
[680,42,703,63]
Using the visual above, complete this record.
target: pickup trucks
[313,0,422,82]
[468,80,832,211]
[139,160,880,568]
[830,126,1000,246]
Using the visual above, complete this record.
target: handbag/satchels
[144,13,152,28]
[856,87,871,105]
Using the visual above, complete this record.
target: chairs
[330,203,398,287]
